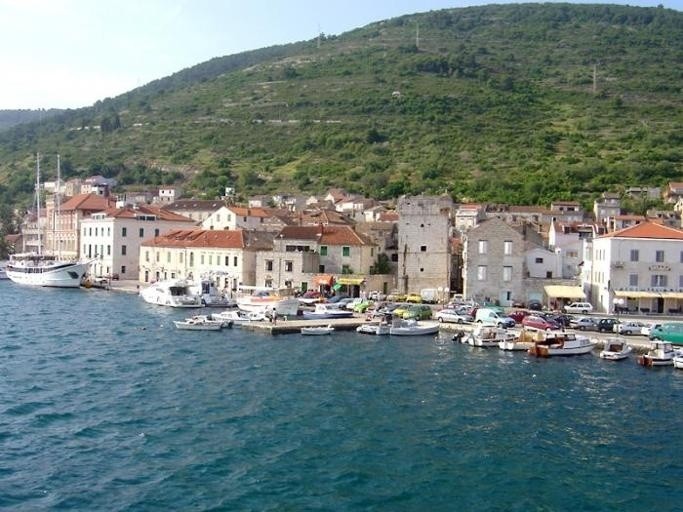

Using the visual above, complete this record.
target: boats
[0,267,10,280]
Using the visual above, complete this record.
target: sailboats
[3,150,102,290]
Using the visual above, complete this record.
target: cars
[105,272,119,280]
[93,274,108,284]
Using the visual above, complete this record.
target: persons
[271,307,277,326]
